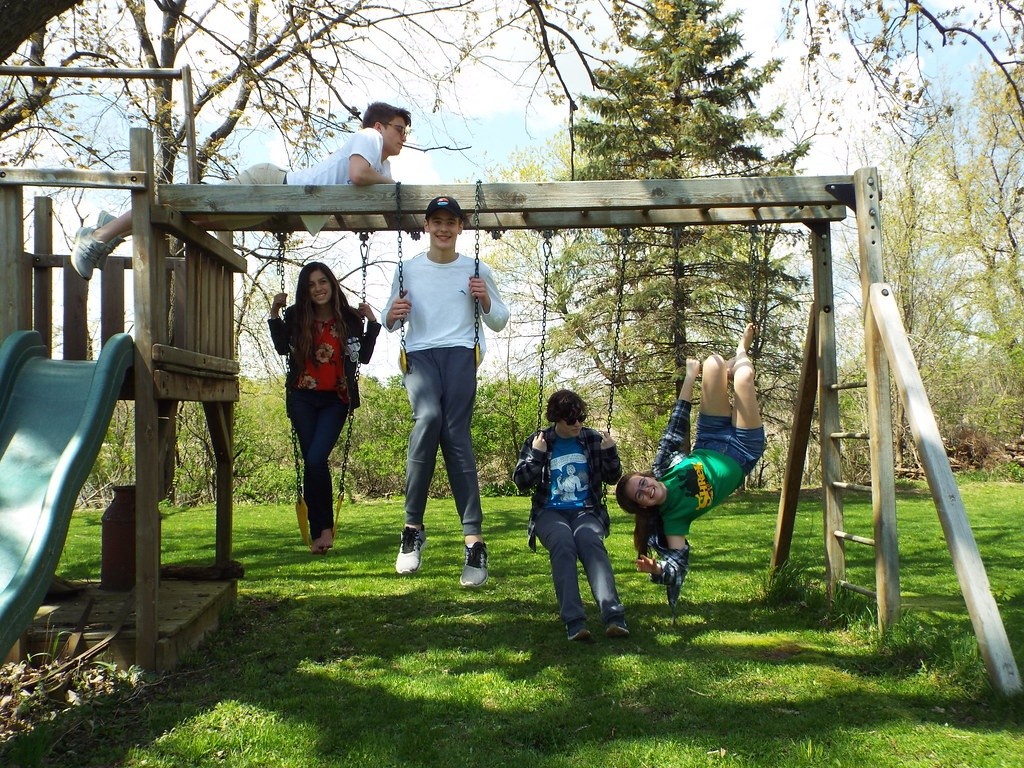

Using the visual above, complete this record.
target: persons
[513,389,628,640]
[70,103,411,282]
[381,197,511,588]
[616,323,766,585]
[270,262,381,555]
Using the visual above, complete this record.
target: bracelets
[372,319,377,323]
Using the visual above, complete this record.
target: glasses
[557,413,587,426]
[381,121,408,138]
[635,476,648,504]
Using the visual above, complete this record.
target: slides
[0,327,134,674]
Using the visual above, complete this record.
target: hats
[425,196,464,220]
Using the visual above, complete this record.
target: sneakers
[395,524,427,575]
[565,615,630,642]
[459,542,489,587]
[70,227,106,281]
[94,211,128,273]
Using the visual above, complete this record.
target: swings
[277,229,369,554]
[537,226,628,513]
[671,224,758,458]
[396,181,481,375]
